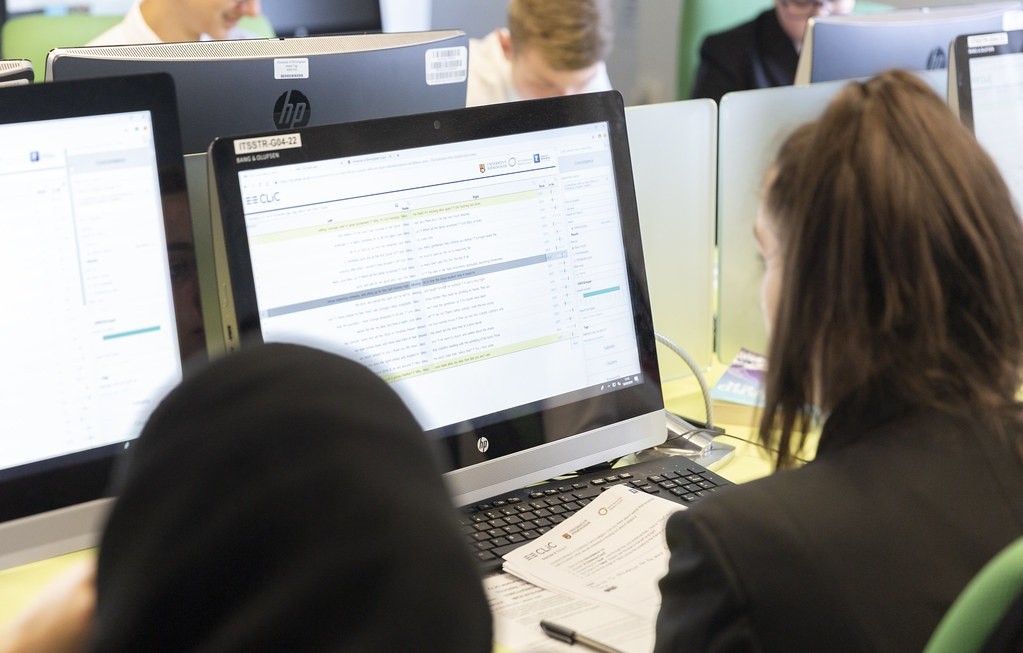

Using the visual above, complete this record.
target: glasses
[778,0,856,15]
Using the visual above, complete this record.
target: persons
[0,343,494,653]
[695,0,855,100]
[648,69,1023,653]
[465,0,612,110]
[82,0,262,46]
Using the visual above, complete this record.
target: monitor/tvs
[790,4,1023,219]
[0,56,205,579]
[47,28,471,159]
[206,90,669,508]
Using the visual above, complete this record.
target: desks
[0,350,824,653]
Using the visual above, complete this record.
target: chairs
[926,537,1023,653]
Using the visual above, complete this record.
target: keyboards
[452,453,740,575]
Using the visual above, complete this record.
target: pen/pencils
[540,620,624,653]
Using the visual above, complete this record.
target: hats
[94,342,493,653]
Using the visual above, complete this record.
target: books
[483,485,690,653]
[708,348,820,432]
